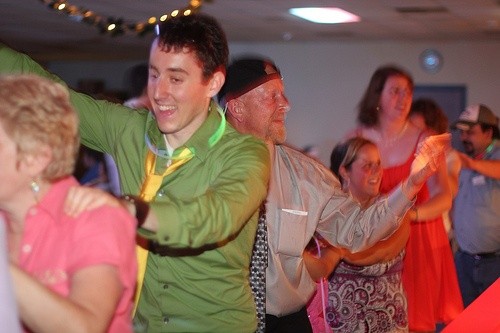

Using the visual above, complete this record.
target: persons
[435,104,500,333]
[315,138,410,333]
[0,13,272,333]
[408,99,462,253]
[342,66,464,333]
[303,232,345,333]
[0,76,137,333]
[218,59,451,333]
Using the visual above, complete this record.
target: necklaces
[372,120,410,143]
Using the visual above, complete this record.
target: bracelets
[414,207,419,223]
[120,194,150,229]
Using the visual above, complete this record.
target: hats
[217,54,283,104]
[450,102,497,131]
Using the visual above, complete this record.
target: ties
[248,211,268,333]
[131,149,194,319]
